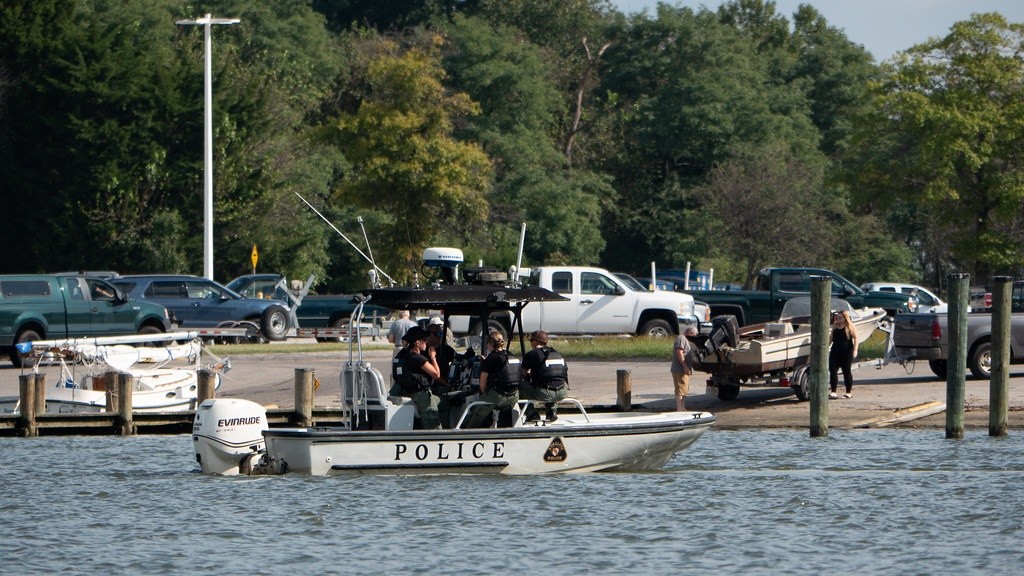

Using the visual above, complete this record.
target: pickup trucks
[473,264,949,344]
[0,266,391,367]
[896,278,1023,380]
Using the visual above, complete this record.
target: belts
[493,387,517,396]
[538,385,563,391]
[395,345,404,347]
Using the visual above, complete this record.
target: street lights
[175,10,243,294]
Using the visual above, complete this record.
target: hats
[401,327,431,340]
[427,317,443,327]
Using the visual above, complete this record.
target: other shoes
[844,393,852,398]
[829,392,837,399]
[526,412,540,421]
[546,409,557,421]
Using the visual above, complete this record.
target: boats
[687,296,887,401]
[190,293,717,478]
[9,329,232,421]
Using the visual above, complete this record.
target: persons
[670,326,697,413]
[387,310,570,431]
[78,270,114,300]
[828,310,859,399]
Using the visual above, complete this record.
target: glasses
[431,332,443,337]
[529,339,539,343]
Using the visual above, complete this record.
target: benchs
[338,366,422,432]
